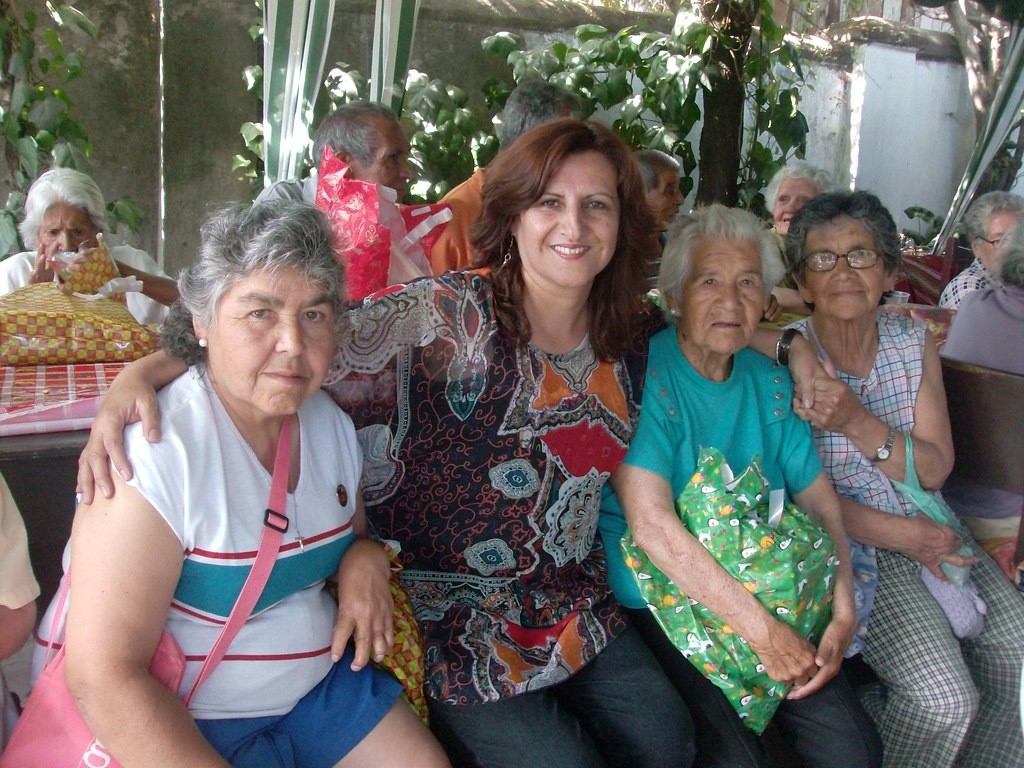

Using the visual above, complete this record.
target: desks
[0,358,158,631]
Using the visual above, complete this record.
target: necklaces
[208,375,306,552]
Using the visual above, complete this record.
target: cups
[884,290,911,305]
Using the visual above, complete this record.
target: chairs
[937,357,1024,494]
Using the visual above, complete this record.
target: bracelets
[775,327,801,366]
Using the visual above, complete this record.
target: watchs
[870,427,896,462]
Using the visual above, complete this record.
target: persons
[628,151,781,323]
[251,100,415,209]
[0,168,180,328]
[426,80,581,276]
[940,189,1024,311]
[781,191,1023,768]
[27,196,453,768]
[75,117,833,768]
[939,222,1023,516]
[0,475,41,757]
[761,160,835,321]
[596,202,884,768]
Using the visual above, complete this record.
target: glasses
[800,247,886,273]
[974,234,1010,250]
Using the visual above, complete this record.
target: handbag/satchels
[885,431,988,639]
[0,622,187,768]
[0,239,157,365]
[619,446,837,737]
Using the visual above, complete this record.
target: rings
[37,278,40,281]
